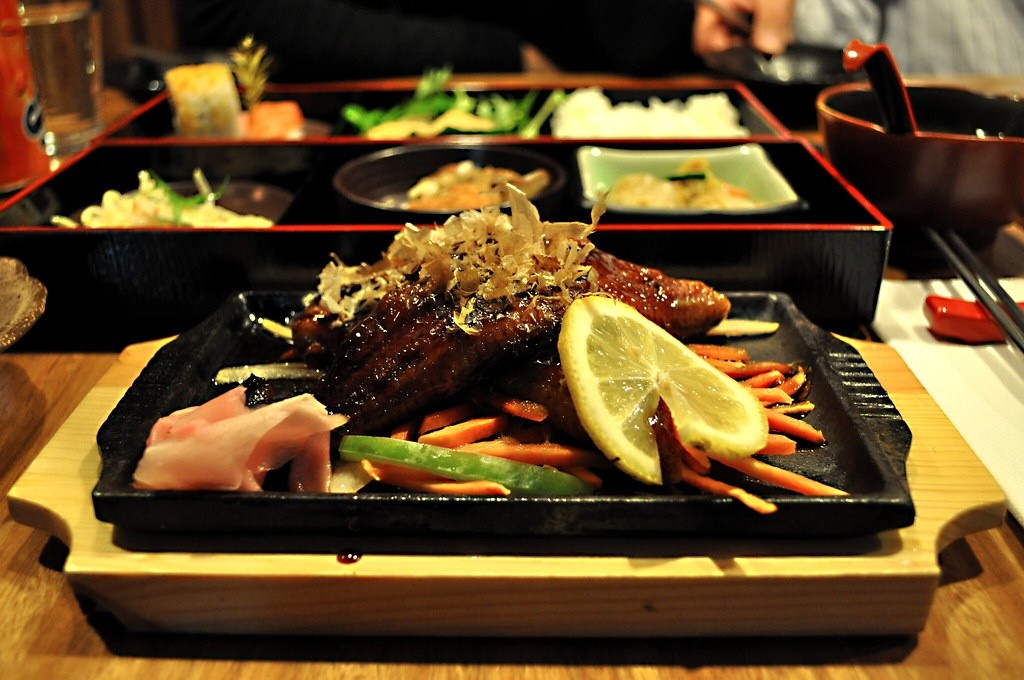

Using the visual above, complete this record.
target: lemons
[559,296,768,483]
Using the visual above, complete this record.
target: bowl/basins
[576,142,809,222]
[815,74,1024,277]
[334,143,565,221]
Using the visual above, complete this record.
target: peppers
[335,434,596,497]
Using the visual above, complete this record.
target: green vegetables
[341,68,562,137]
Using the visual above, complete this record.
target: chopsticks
[927,226,1024,353]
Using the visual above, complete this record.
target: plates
[90,286,916,545]
[117,182,293,222]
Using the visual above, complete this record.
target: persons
[695,0,1024,80]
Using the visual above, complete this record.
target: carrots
[369,343,850,512]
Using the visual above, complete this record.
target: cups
[13,1,108,157]
